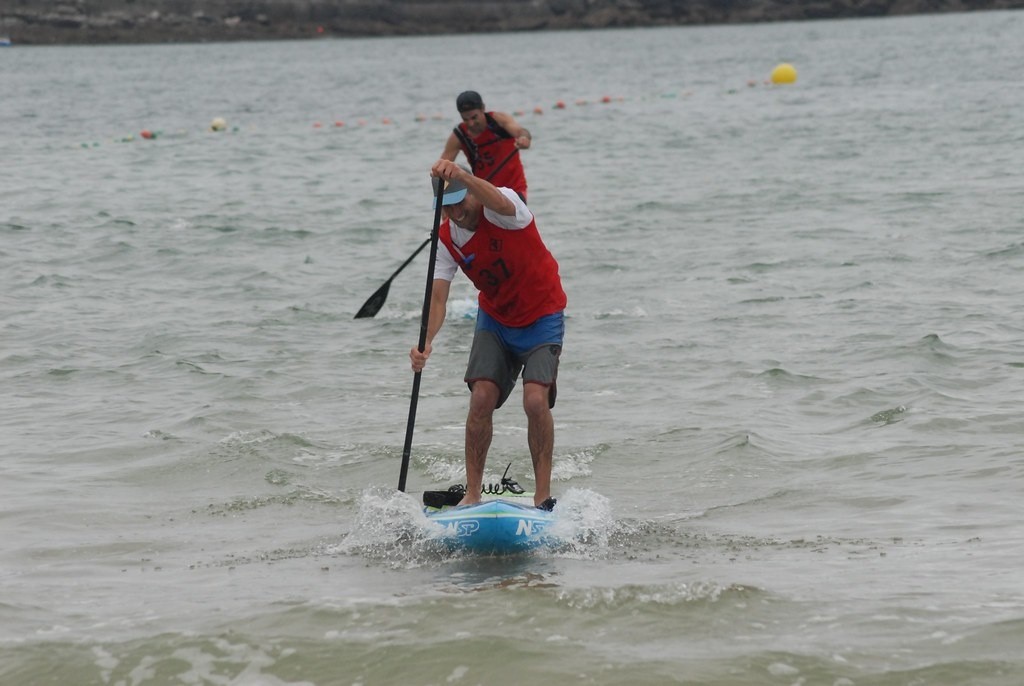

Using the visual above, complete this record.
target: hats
[457,90,484,112]
[431,162,472,209]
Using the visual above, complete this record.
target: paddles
[353,148,519,319]
[397,178,445,492]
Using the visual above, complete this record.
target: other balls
[770,63,797,84]
[211,118,227,131]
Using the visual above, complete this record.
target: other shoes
[538,496,556,514]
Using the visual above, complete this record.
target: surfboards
[422,487,560,553]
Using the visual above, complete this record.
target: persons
[409,159,569,511]
[441,91,531,205]
